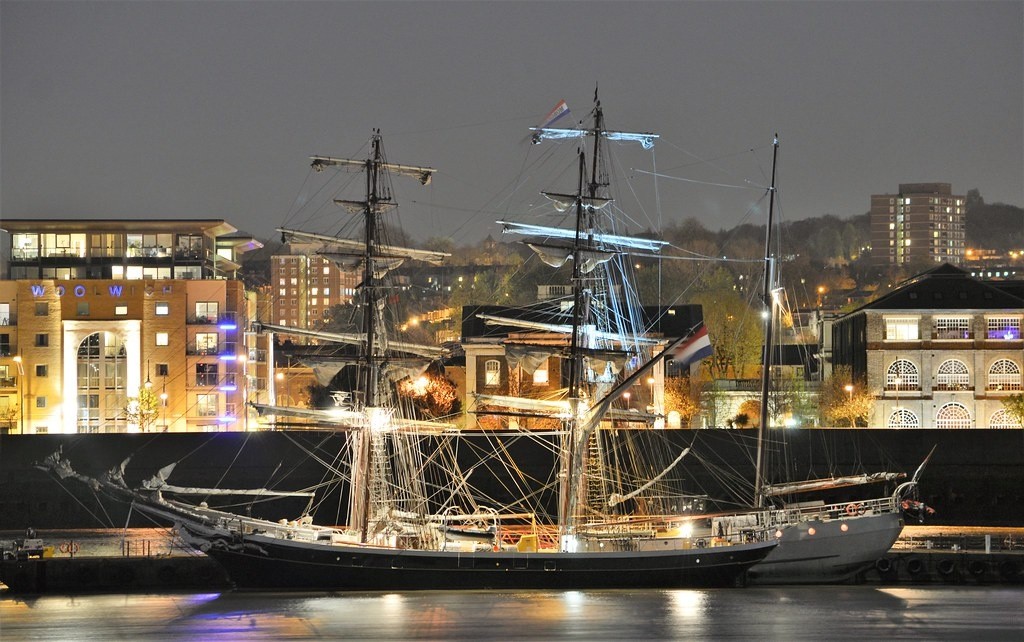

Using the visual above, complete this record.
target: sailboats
[36,78,935,596]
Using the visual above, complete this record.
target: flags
[677,325,714,366]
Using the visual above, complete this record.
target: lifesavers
[1000,560,1016,576]
[198,566,213,580]
[937,558,955,574]
[159,564,177,581]
[968,560,985,574]
[876,556,891,571]
[846,504,858,516]
[60,542,70,553]
[67,543,79,553]
[856,503,866,515]
[906,557,922,573]
